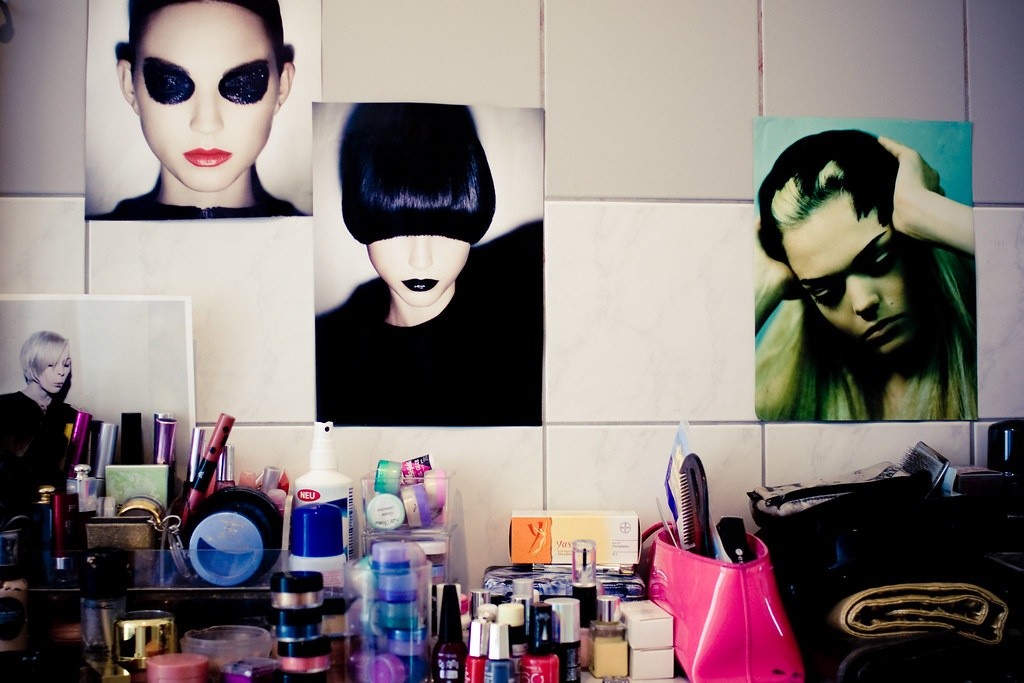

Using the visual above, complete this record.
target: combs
[678,453,716,559]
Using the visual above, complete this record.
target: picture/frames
[0,293,194,504]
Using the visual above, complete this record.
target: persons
[755,129,979,419]
[85,0,310,221]
[0,332,85,493]
[315,103,546,423]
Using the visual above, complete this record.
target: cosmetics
[0,412,673,683]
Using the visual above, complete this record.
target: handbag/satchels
[642,519,805,683]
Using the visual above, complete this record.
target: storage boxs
[507,511,676,681]
